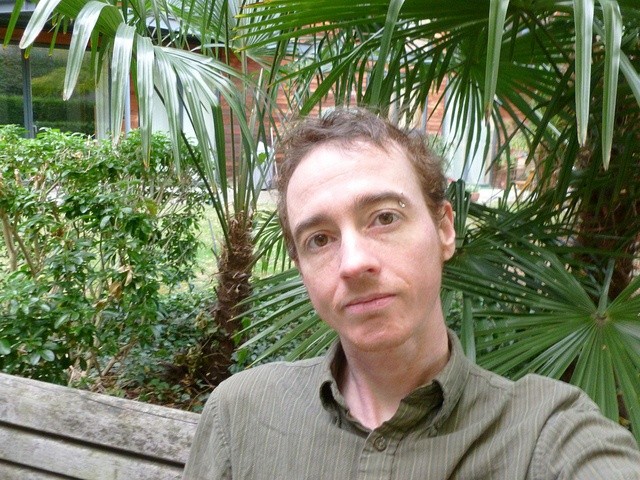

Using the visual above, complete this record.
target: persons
[181,108,639,479]
